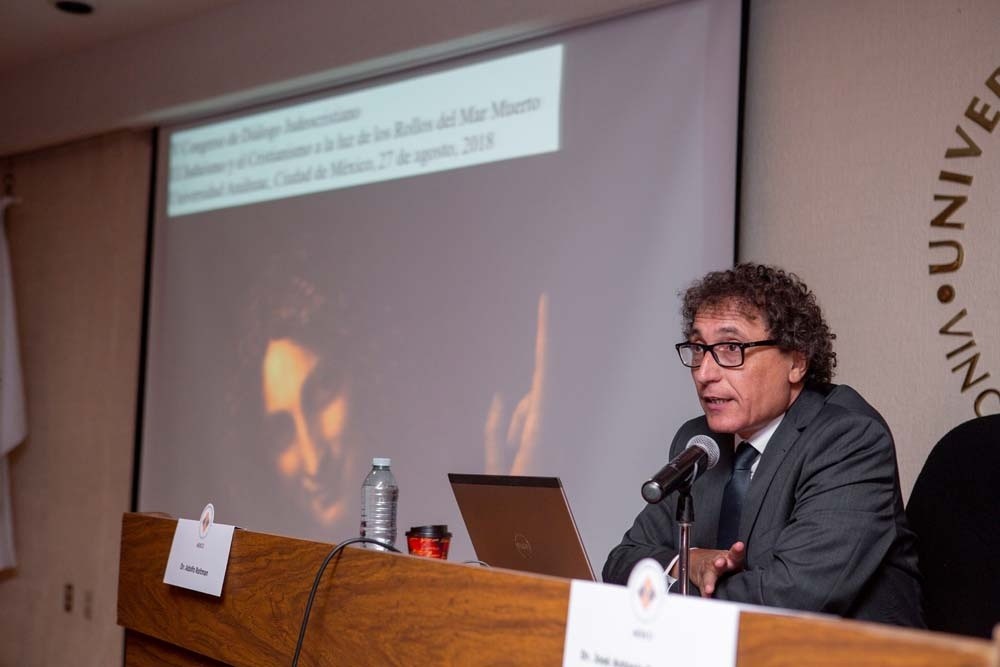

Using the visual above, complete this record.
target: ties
[716,440,761,551]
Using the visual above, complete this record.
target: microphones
[641,435,720,505]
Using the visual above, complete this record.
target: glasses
[676,338,781,368]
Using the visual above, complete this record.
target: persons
[602,265,924,627]
[231,279,547,535]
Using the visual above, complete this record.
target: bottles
[361,458,399,552]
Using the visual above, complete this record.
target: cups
[404,524,452,559]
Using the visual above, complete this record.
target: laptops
[446,473,598,584]
